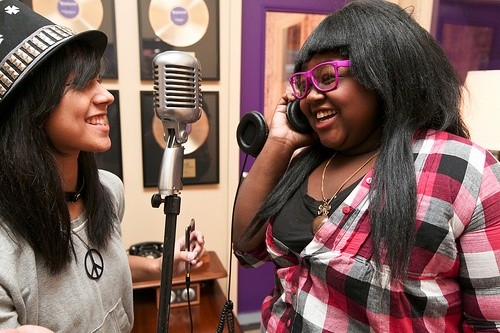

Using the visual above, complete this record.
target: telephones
[129,241,164,259]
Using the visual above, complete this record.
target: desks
[131,279,244,333]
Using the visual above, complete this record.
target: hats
[0,0,108,102]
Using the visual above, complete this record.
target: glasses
[289,59,350,99]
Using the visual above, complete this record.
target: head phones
[235,100,315,159]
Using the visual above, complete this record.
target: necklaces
[71,218,104,280]
[311,146,380,234]
[63,174,86,203]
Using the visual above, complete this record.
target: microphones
[151,51,203,200]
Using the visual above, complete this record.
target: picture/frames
[140,91,221,188]
[93,90,123,180]
[137,0,221,81]
[18,1,119,80]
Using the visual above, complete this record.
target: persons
[0,1,206,333]
[232,0,500,333]
